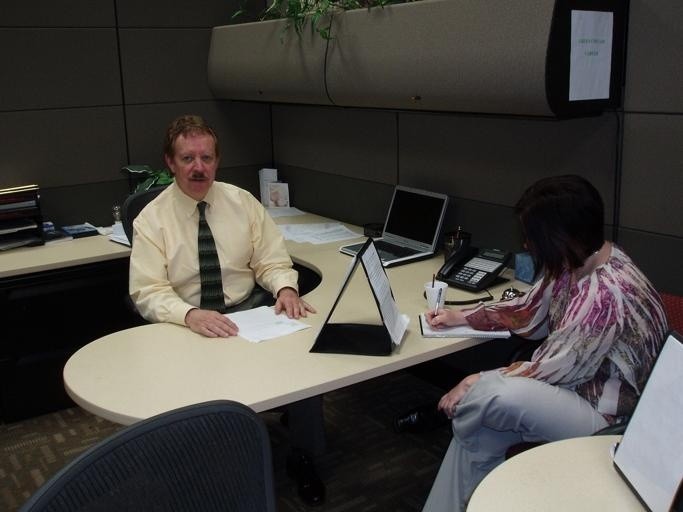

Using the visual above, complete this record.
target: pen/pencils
[447,224,464,253]
[432,289,442,330]
[432,274,435,288]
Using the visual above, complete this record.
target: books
[417,312,511,339]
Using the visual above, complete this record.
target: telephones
[436,245,511,292]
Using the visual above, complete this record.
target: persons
[392,173,668,512]
[128,114,326,507]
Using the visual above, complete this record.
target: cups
[423,281,448,311]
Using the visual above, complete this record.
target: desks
[463,433,653,512]
[0,207,541,512]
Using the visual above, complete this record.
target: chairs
[505,421,628,458]
[121,183,171,246]
[17,399,275,512]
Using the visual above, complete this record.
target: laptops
[339,183,450,267]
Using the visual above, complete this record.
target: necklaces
[570,249,598,286]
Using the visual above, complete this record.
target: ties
[197,201,228,313]
